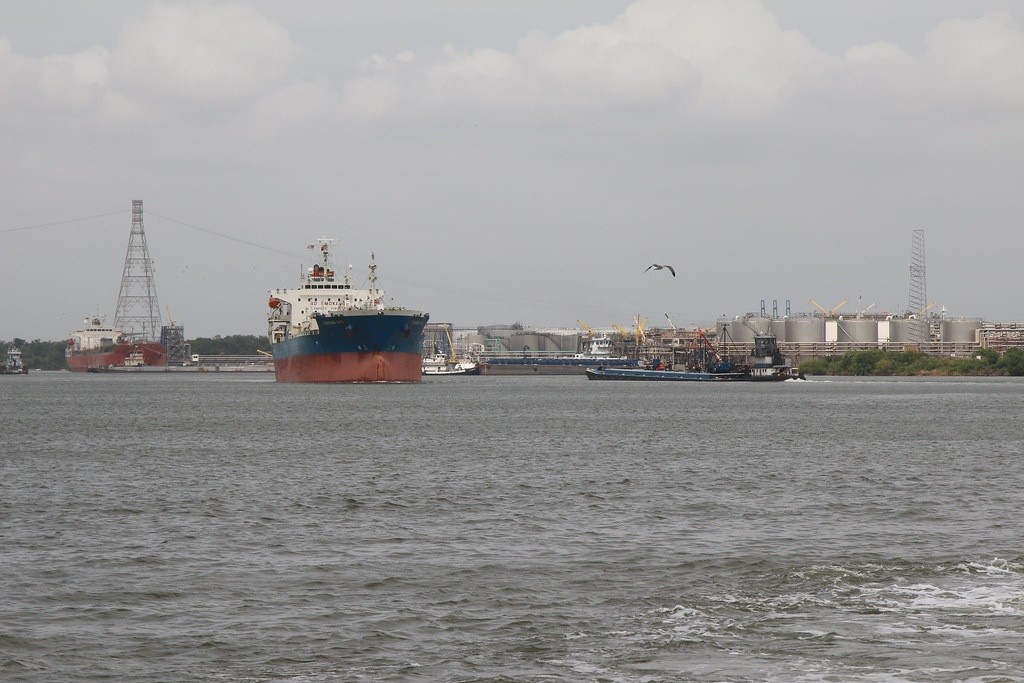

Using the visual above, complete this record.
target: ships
[64,306,167,373]
[266,237,431,385]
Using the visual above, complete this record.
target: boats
[0,344,29,376]
[420,352,478,376]
[584,334,807,381]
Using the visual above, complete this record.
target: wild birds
[640,264,676,278]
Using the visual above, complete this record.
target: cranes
[665,313,677,337]
[574,313,647,346]
[809,294,878,318]
